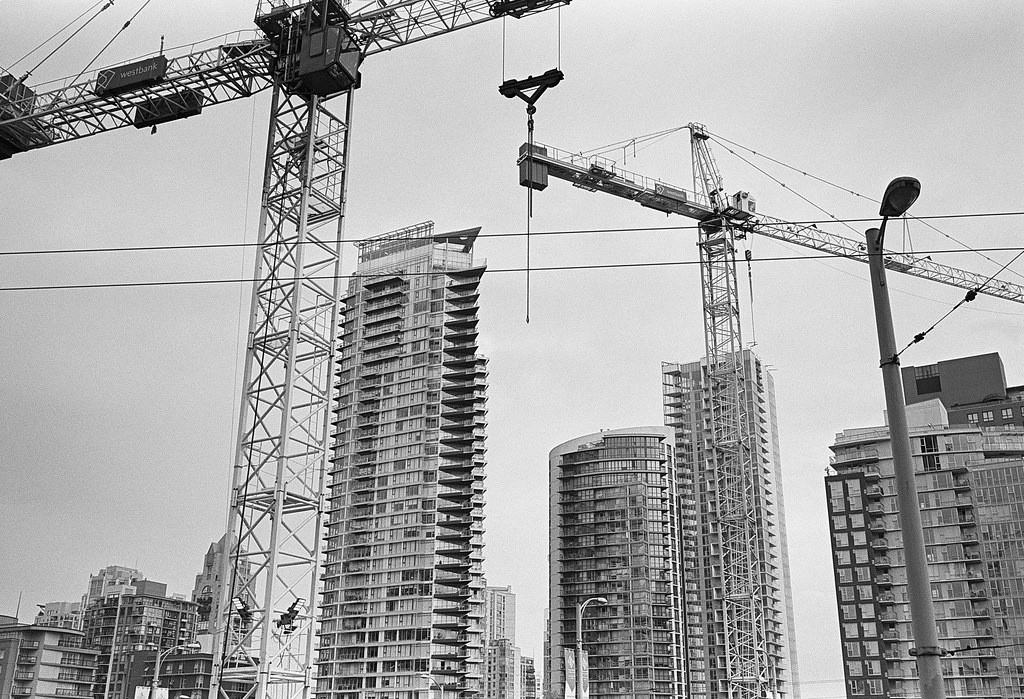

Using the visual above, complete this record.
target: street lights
[866,176,948,699]
[575,597,607,699]
[152,642,201,698]
[421,674,443,699]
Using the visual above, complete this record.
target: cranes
[518,121,1024,699]
[0,0,572,699]
